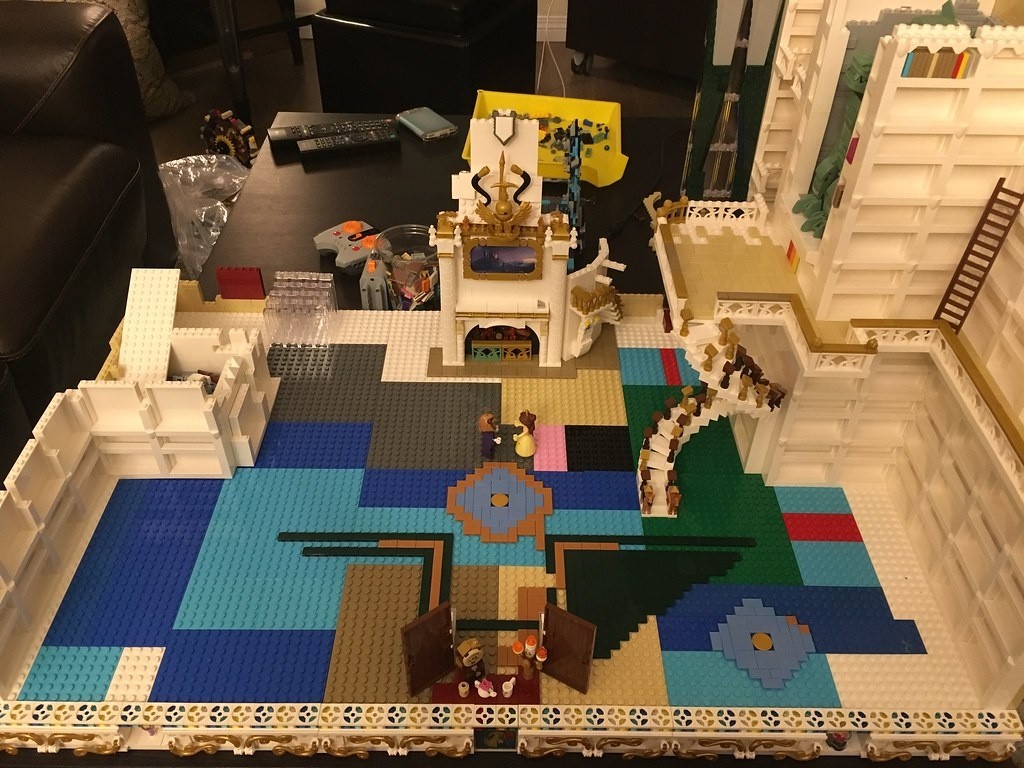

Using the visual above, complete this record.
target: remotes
[266,118,400,146]
[296,129,400,154]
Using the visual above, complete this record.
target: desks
[195,109,695,294]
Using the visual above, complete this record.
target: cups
[373,224,439,311]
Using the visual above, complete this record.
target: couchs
[0,0,181,493]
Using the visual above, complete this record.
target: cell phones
[395,106,459,142]
